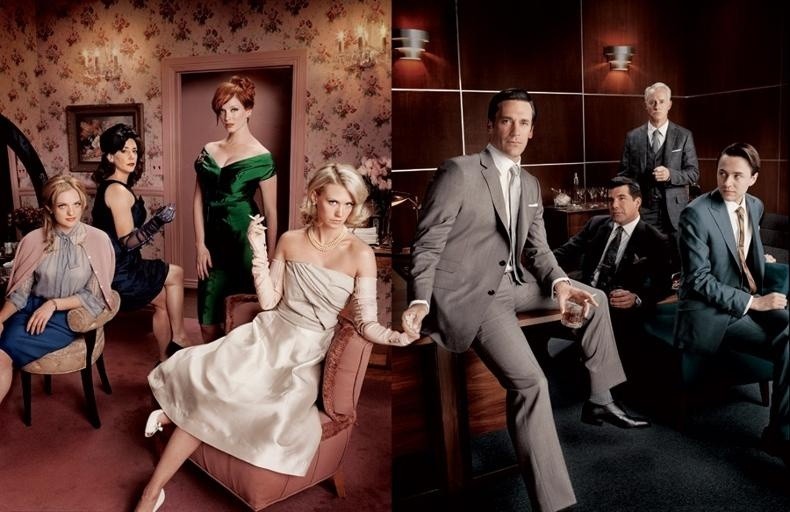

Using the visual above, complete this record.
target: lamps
[391,190,422,226]
[339,24,388,70]
[82,47,121,81]
[603,44,635,70]
[392,29,429,60]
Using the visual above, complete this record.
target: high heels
[167,341,183,358]
[154,491,165,512]
[144,410,166,439]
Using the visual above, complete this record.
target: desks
[368,240,393,369]
[542,203,610,246]
[413,307,568,497]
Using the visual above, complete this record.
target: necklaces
[307,225,349,252]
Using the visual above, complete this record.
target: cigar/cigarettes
[583,293,598,303]
[168,205,175,214]
[246,213,255,221]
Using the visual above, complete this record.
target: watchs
[667,170,673,183]
[632,293,642,307]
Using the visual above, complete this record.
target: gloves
[246,215,283,309]
[350,278,419,346]
[116,203,179,252]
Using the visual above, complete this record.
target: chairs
[567,266,678,369]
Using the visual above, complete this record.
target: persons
[92,124,194,367]
[402,89,651,511]
[552,177,667,308]
[0,175,114,405]
[617,83,699,230]
[194,76,277,339]
[135,164,420,512]
[679,142,789,460]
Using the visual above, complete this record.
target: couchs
[19,289,121,428]
[759,211,790,264]
[154,294,374,512]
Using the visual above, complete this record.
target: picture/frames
[65,103,145,173]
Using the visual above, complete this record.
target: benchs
[645,339,774,429]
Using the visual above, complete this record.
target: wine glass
[571,187,609,209]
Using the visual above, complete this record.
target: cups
[562,300,583,329]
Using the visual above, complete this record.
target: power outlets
[150,159,163,175]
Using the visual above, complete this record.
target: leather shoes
[583,401,653,430]
[758,427,786,459]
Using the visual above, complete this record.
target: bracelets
[50,298,60,312]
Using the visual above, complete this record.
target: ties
[595,225,625,289]
[652,129,662,151]
[509,167,526,286]
[737,208,757,293]
